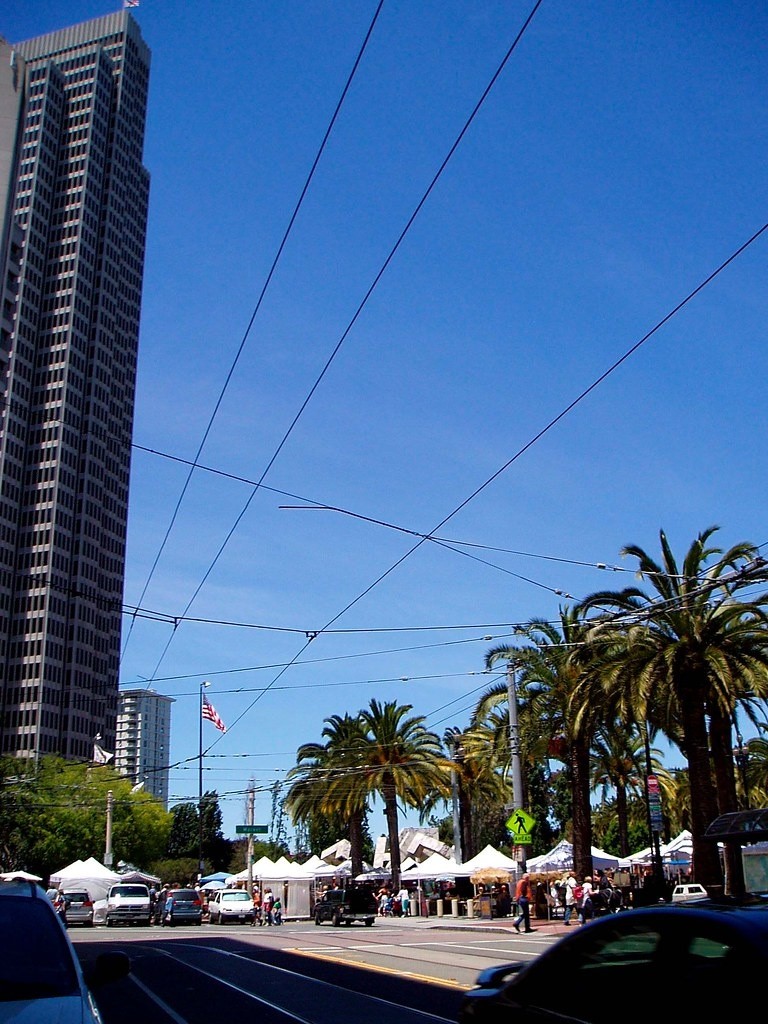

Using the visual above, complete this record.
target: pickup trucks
[207,889,255,925]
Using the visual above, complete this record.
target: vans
[164,888,203,926]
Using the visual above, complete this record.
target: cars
[0,875,102,1024]
[464,903,768,1024]
[672,883,709,904]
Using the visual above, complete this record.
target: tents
[526,829,744,873]
[200,845,521,884]
[0,857,162,901]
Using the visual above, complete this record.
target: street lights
[448,743,466,867]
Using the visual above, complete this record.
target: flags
[93,745,113,764]
[202,693,226,731]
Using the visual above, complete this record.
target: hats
[58,889,63,892]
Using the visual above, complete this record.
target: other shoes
[513,923,519,932]
[564,922,571,925]
[525,927,537,932]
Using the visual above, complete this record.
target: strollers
[595,887,623,916]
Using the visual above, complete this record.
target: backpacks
[573,886,588,899]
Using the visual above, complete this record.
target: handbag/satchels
[519,896,529,906]
[165,913,171,921]
[65,900,71,910]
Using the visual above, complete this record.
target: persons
[311,876,510,919]
[149,881,283,927]
[47,886,68,930]
[513,865,691,933]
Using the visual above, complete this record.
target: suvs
[312,887,379,927]
[105,884,152,929]
[60,889,96,928]
